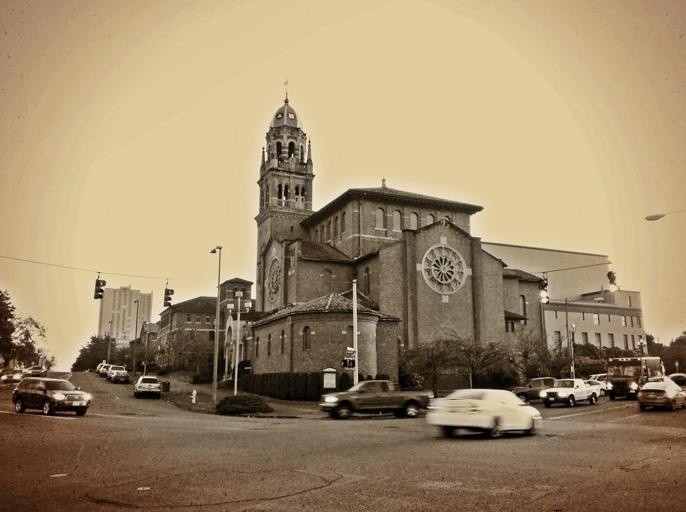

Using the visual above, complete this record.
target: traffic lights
[163,288,174,307]
[93,278,106,300]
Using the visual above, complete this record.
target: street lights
[132,299,139,376]
[209,244,222,402]
[107,319,113,364]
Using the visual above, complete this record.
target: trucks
[606,356,665,400]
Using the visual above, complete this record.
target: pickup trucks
[318,378,434,418]
[540,378,601,408]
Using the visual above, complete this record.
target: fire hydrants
[190,390,197,404]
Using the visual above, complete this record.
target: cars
[96,363,129,383]
[427,388,543,439]
[11,377,92,416]
[585,374,607,397]
[1,370,32,383]
[638,373,686,412]
[22,365,47,377]
[511,376,558,403]
[134,375,161,399]
